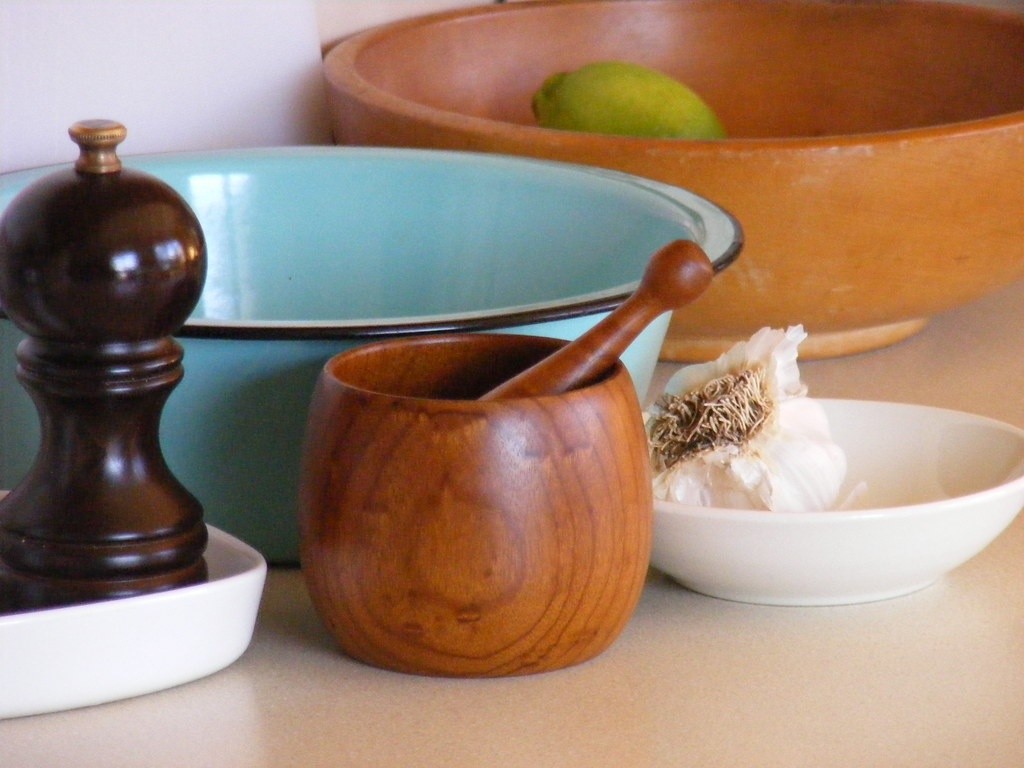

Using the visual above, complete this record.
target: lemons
[530,56,730,140]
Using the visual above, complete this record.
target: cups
[297,328,653,677]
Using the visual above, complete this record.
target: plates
[649,395,1023,608]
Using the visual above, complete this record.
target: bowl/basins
[2,144,745,571]
[323,1,1023,361]
